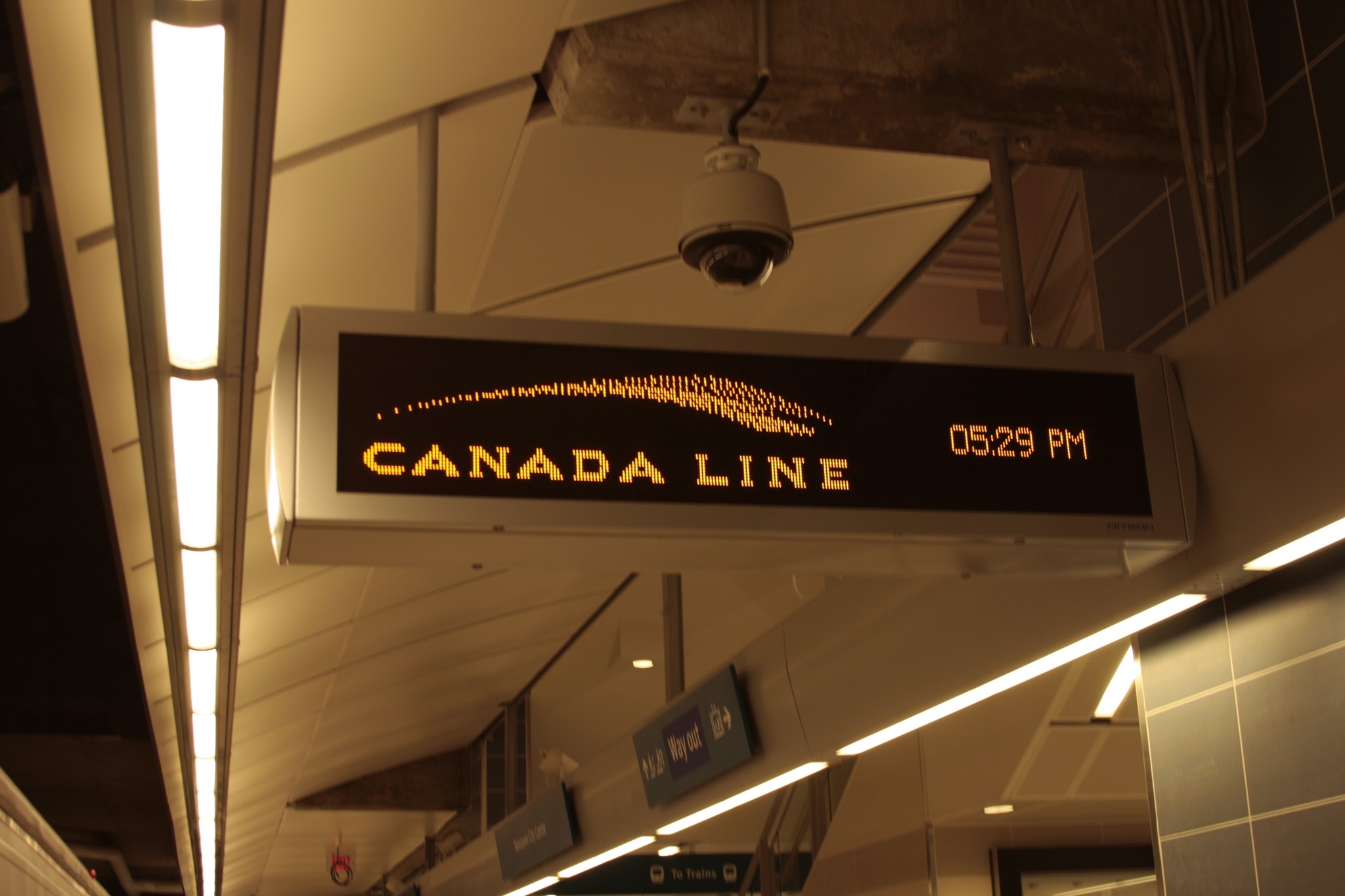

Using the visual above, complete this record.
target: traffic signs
[632,663,758,808]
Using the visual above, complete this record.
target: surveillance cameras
[678,169,795,293]
